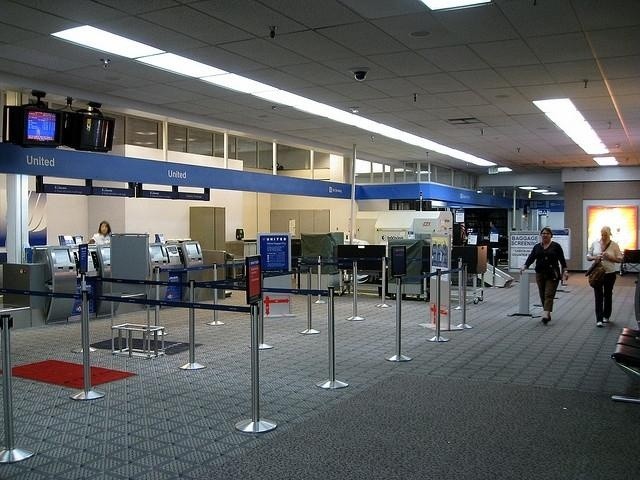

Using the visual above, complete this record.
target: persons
[488,222,499,237]
[586,226,624,328]
[518,227,570,325]
[86,220,113,267]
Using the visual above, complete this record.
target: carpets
[0,336,203,390]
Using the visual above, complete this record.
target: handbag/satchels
[551,265,562,281]
[585,259,606,288]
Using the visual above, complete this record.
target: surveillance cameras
[349,68,369,81]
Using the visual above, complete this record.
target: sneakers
[541,316,551,326]
[596,321,603,327]
[603,316,609,322]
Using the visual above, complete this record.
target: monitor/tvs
[54,249,71,264]
[9,106,62,149]
[187,245,199,255]
[103,247,110,265]
[65,113,116,153]
[454,212,464,224]
[149,246,164,259]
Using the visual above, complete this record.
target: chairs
[610,328,639,402]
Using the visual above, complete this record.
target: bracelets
[563,270,568,273]
